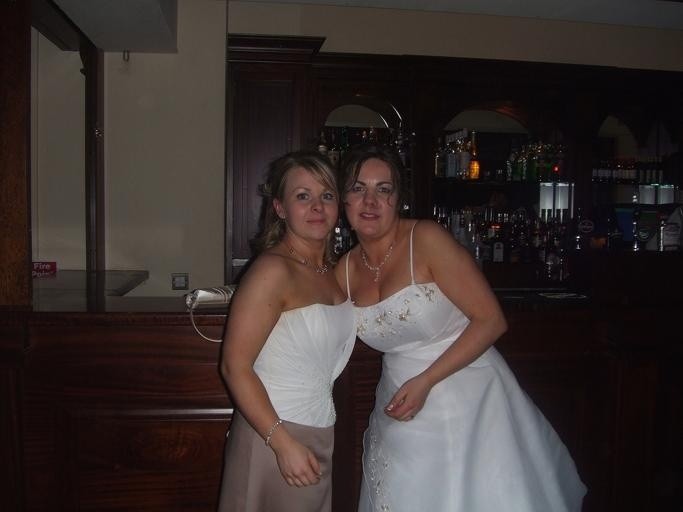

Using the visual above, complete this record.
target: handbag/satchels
[185,285,236,310]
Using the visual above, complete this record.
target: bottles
[432,127,665,282]
[318,121,408,169]
[333,217,350,258]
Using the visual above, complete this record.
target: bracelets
[265,420,282,446]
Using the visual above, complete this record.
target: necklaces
[360,242,393,282]
[284,239,328,273]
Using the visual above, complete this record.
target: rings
[411,415,414,419]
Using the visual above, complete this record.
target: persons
[333,144,588,512]
[217,152,358,512]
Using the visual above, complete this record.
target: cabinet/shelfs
[318,174,576,288]
